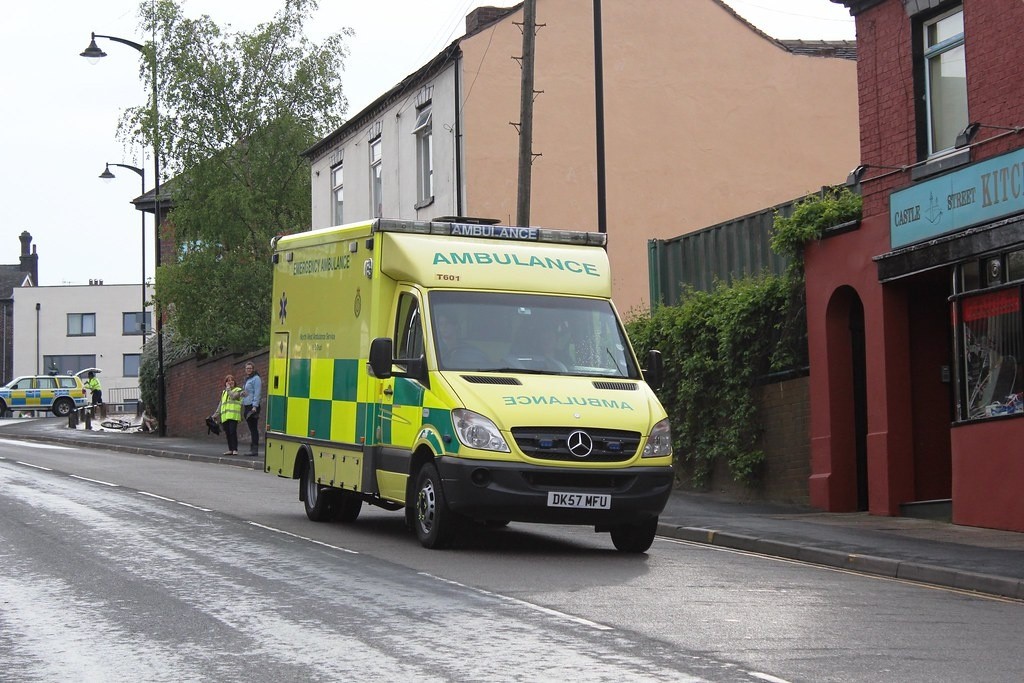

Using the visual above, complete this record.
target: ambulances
[266,214,673,554]
[0,368,102,417]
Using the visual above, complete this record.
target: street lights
[79,32,163,435]
[99,158,148,411]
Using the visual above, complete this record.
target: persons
[83,371,104,404]
[212,375,243,456]
[428,310,471,353]
[241,361,262,456]
[141,409,158,433]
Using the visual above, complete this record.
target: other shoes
[223,451,232,455]
[245,451,258,456]
[233,450,237,454]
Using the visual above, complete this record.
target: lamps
[845,164,906,187]
[955,122,1019,149]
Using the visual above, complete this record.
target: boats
[100,418,131,433]
